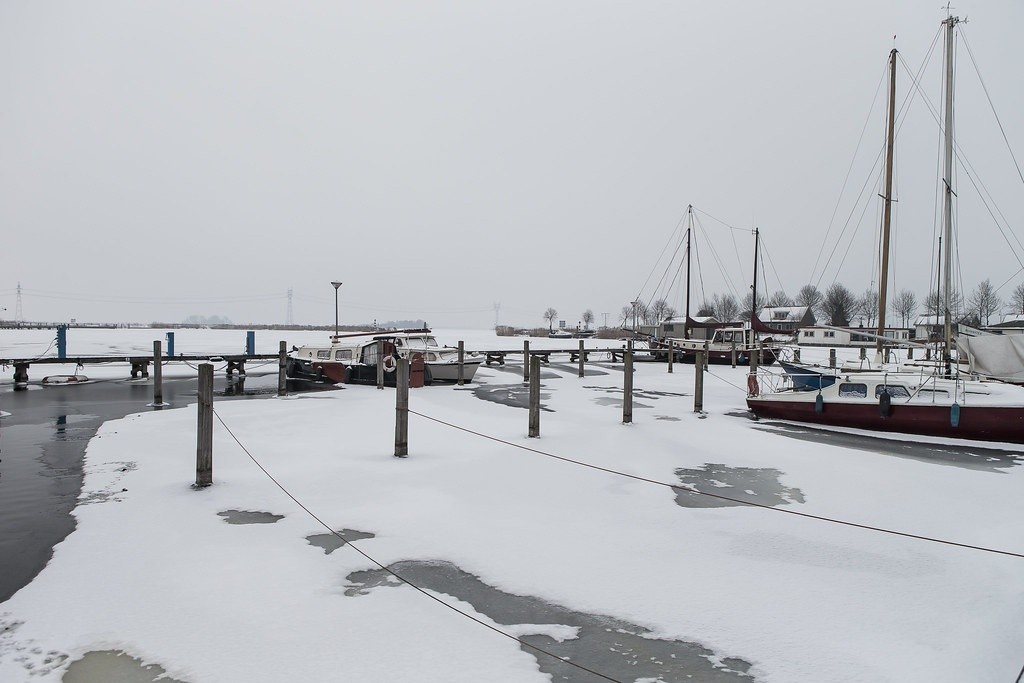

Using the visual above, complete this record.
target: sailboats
[623,9,1024,440]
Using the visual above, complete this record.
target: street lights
[330,281,343,336]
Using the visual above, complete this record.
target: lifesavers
[383,355,397,372]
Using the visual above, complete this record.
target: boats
[287,325,485,383]
